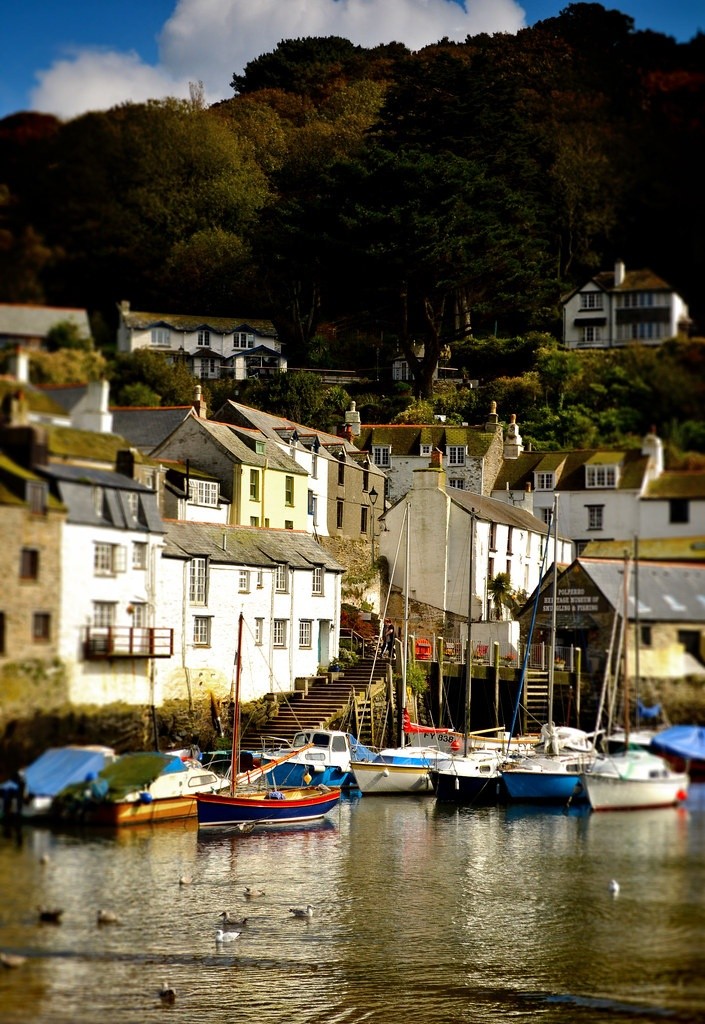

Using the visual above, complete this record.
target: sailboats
[200,614,343,838]
[266,490,704,815]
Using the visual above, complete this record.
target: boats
[0,740,234,824]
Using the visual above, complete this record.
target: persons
[386,620,395,658]
[380,619,391,657]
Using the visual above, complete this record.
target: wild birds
[219,912,248,923]
[179,873,194,883]
[0,952,27,967]
[608,878,620,891]
[37,903,66,922]
[288,904,316,916]
[215,930,242,942]
[159,981,177,1000]
[97,908,118,923]
[40,850,50,864]
[244,887,266,896]
